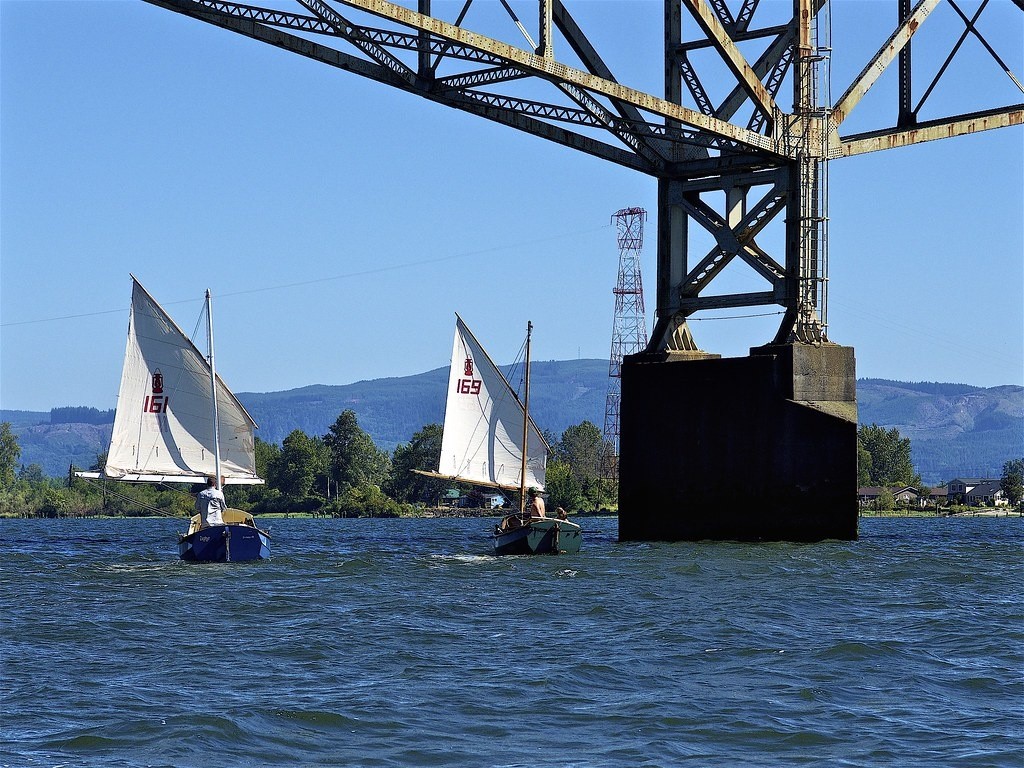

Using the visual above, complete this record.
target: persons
[195,475,227,528]
[555,506,568,522]
[528,487,545,522]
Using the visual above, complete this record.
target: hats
[528,487,537,494]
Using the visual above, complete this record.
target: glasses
[222,484,225,486]
[558,511,563,514]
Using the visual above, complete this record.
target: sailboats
[73,272,276,565]
[409,308,583,560]
